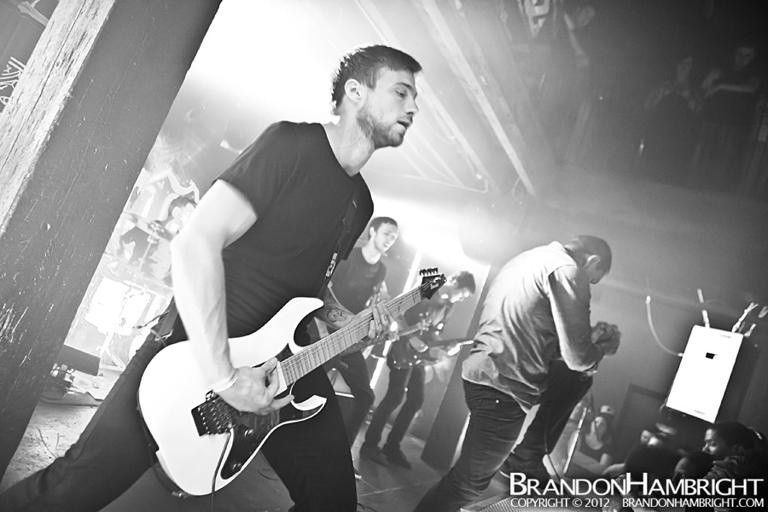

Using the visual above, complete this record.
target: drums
[85,273,151,336]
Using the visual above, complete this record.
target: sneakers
[382,441,411,469]
[360,442,391,467]
[499,453,565,492]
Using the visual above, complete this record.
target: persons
[359,269,476,469]
[1,44,422,512]
[408,234,621,511]
[565,397,767,511]
[69,194,205,406]
[321,215,400,483]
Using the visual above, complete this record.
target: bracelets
[211,367,240,398]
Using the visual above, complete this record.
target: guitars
[137,268,447,503]
[387,335,475,371]
